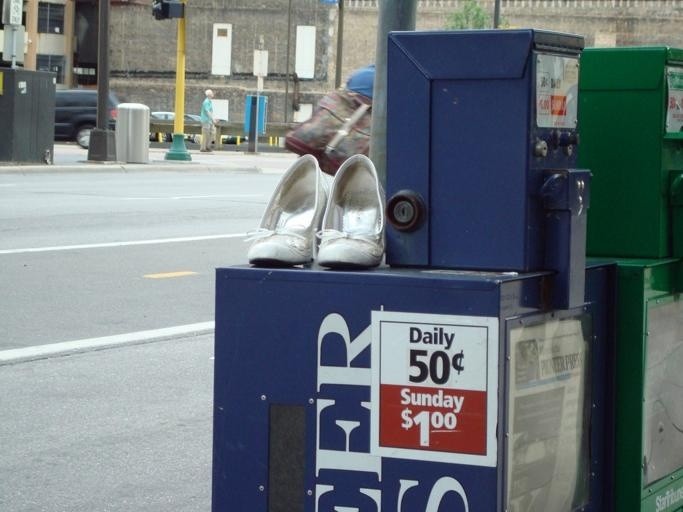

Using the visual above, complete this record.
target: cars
[148,111,201,146]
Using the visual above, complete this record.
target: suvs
[53,90,119,148]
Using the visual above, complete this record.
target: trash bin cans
[115,103,150,164]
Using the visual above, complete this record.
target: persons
[199,88,218,151]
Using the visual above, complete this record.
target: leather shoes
[248,152,329,265]
[317,154,385,268]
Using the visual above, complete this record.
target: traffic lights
[151,0,183,21]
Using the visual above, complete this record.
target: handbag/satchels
[285,88,372,176]
